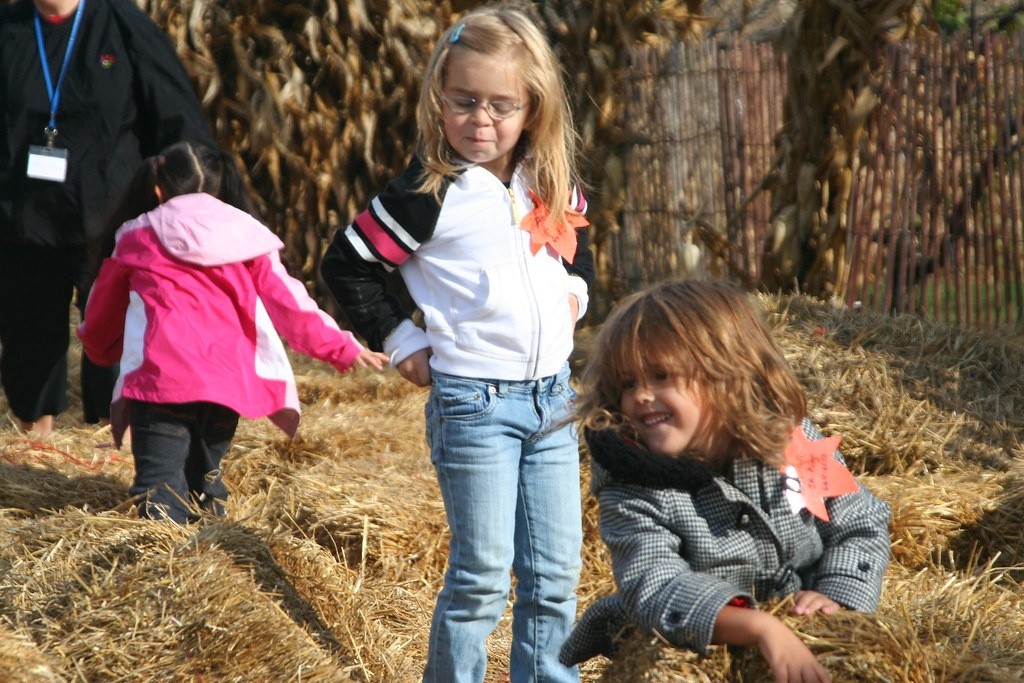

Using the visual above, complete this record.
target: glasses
[442,96,533,119]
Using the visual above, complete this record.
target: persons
[0,0,218,435]
[319,7,597,683]
[74,138,392,525]
[557,269,891,683]
